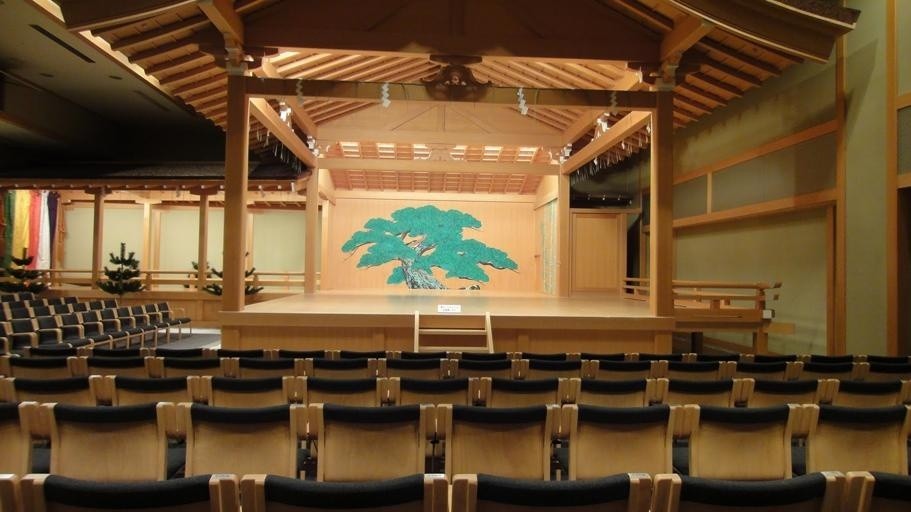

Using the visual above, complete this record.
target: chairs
[21,472,240,512]
[85,346,150,357]
[28,298,46,307]
[103,299,118,309]
[2,300,28,308]
[17,292,35,301]
[825,378,911,407]
[449,359,520,379]
[150,348,210,357]
[519,359,589,379]
[305,358,378,376]
[98,307,143,350]
[114,307,156,348]
[143,304,180,343]
[52,304,71,314]
[240,473,449,512]
[46,298,64,305]
[684,403,802,482]
[4,374,104,407]
[0,400,39,474]
[656,377,743,407]
[296,376,389,406]
[802,353,860,362]
[0,294,17,303]
[480,377,569,408]
[200,375,295,407]
[689,353,746,361]
[308,403,436,482]
[2,356,79,378]
[88,300,103,311]
[176,401,307,481]
[3,307,32,320]
[79,310,128,350]
[156,301,193,340]
[32,313,94,350]
[32,305,52,317]
[104,375,200,407]
[394,351,454,359]
[801,403,911,475]
[590,359,659,379]
[569,377,656,407]
[436,403,561,484]
[63,296,80,303]
[450,472,653,512]
[210,348,272,358]
[794,361,860,379]
[155,357,231,377]
[562,402,684,480]
[746,352,802,362]
[844,471,911,512]
[332,350,394,359]
[0,472,22,512]
[454,352,514,359]
[24,346,86,356]
[71,302,88,312]
[79,356,156,378]
[573,352,631,360]
[650,471,847,512]
[378,358,449,378]
[231,357,305,377]
[862,354,911,361]
[389,377,479,406]
[860,361,911,379]
[726,360,794,380]
[129,305,169,348]
[272,349,332,358]
[741,378,826,408]
[658,360,726,381]
[631,352,688,361]
[514,351,572,359]
[55,312,112,350]
[7,317,72,350]
[0,322,35,357]
[39,401,175,481]
